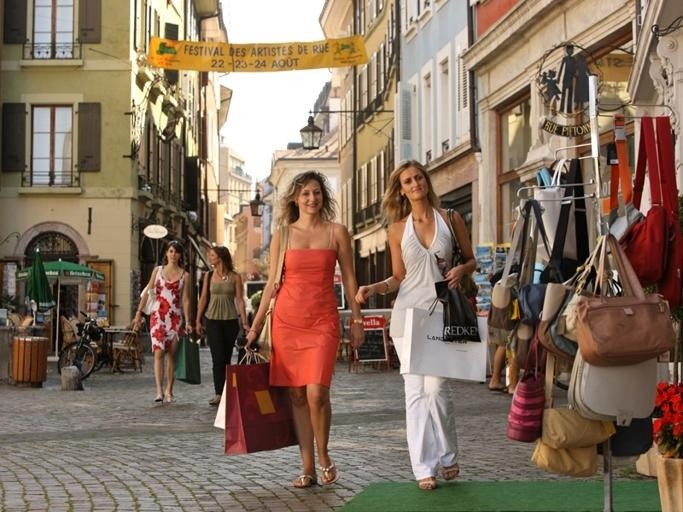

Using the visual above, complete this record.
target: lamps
[199,185,266,217]
[297,106,397,153]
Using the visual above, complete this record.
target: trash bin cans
[13,335,51,388]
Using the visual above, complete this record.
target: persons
[353,159,478,492]
[195,246,251,405]
[135,239,193,403]
[244,169,365,488]
[486,262,521,394]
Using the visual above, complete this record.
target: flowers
[651,389,682,458]
[648,381,681,419]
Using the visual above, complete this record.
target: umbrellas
[14,257,106,356]
[25,247,56,325]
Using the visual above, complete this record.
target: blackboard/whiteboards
[354,328,388,363]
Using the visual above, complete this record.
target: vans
[241,279,268,305]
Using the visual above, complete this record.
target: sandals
[441,463,460,481]
[418,475,437,491]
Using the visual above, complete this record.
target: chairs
[57,305,146,375]
[336,316,354,363]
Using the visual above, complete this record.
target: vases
[653,454,682,512]
[635,416,667,478]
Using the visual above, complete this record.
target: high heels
[154,392,165,404]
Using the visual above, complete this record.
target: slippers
[316,459,341,485]
[292,472,318,488]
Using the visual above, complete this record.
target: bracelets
[378,278,392,296]
[350,318,366,325]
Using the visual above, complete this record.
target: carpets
[337,472,662,512]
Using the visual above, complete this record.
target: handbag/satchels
[433,276,483,345]
[213,347,300,458]
[254,296,276,361]
[452,251,481,298]
[139,285,159,316]
[399,305,490,387]
[173,334,203,386]
[492,113,683,477]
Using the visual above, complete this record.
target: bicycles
[54,310,111,382]
